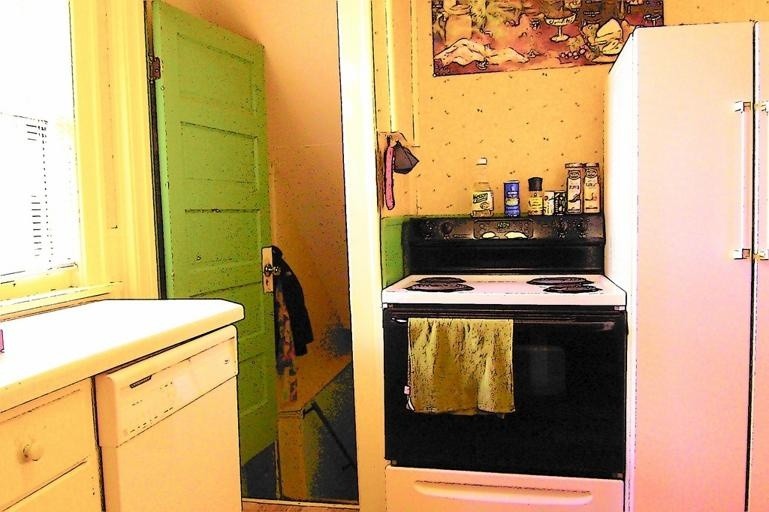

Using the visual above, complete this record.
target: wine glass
[543,12,576,41]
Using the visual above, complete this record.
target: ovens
[385,308,625,479]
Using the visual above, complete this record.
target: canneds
[544,163,601,215]
[504,180,520,217]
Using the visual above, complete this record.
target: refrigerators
[604,23,768,512]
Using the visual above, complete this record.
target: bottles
[566,162,584,215]
[446,5,472,44]
[584,161,602,212]
[527,177,544,215]
[472,158,494,218]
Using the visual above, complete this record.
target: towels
[405,317,517,419]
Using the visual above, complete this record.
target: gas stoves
[407,275,603,293]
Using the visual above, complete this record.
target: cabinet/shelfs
[98,325,242,512]
[2,379,102,510]
[277,356,352,499]
